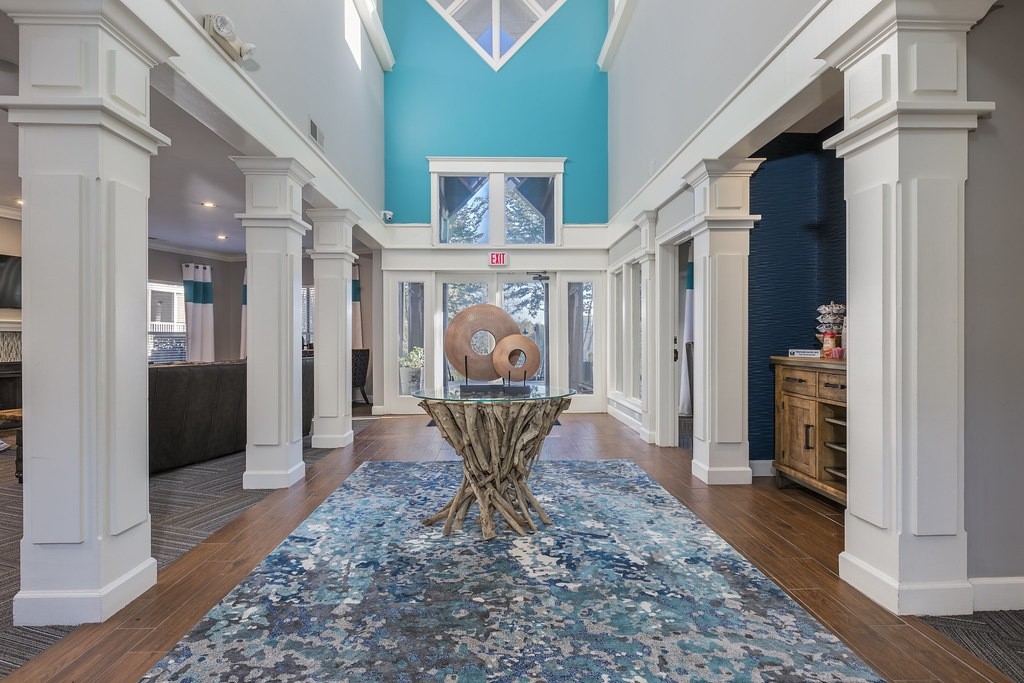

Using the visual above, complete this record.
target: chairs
[352,349,370,404]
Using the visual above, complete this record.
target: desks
[410,385,577,541]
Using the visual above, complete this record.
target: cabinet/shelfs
[770,355,847,508]
